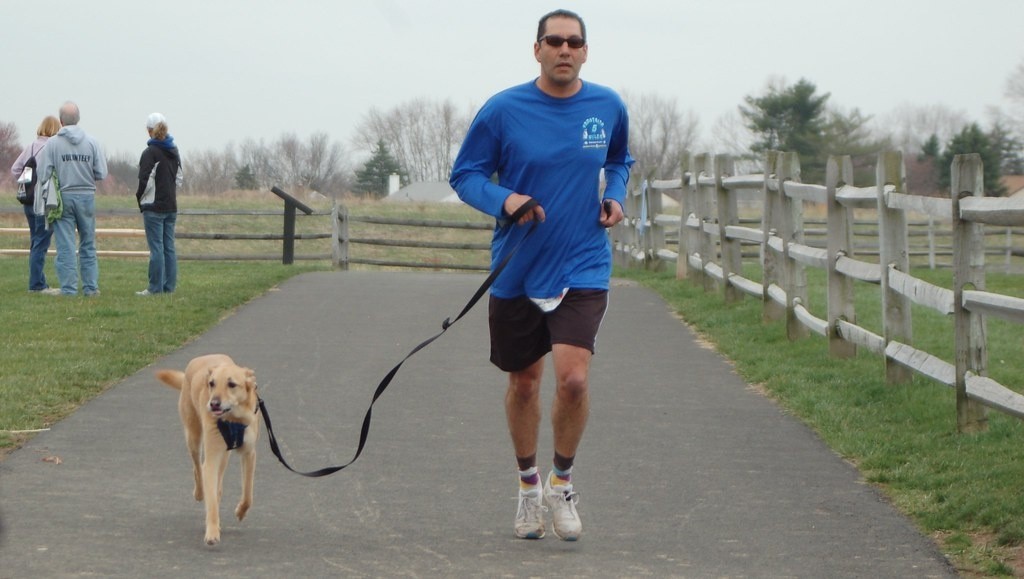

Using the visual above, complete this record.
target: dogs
[160,353,261,545]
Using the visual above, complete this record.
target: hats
[147,113,166,128]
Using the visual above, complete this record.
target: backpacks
[16,142,45,204]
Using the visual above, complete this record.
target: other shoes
[135,289,152,295]
[28,287,60,295]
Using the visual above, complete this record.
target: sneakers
[543,470,582,541]
[515,474,549,539]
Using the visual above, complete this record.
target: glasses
[539,35,585,48]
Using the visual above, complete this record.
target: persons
[10,116,62,296]
[449,9,635,542]
[35,101,108,300]
[136,112,183,296]
